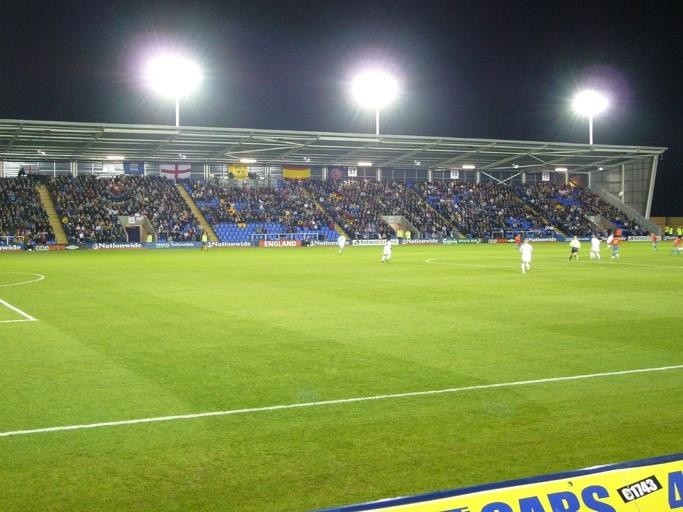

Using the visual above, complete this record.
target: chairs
[407,178,649,240]
[177,175,340,245]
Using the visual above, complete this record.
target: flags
[159,163,190,178]
[228,165,249,178]
[123,163,144,174]
[283,165,310,180]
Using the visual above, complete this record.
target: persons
[670,235,682,256]
[649,232,657,252]
[200,231,207,252]
[568,235,581,263]
[662,221,682,241]
[0,168,203,247]
[336,232,346,254]
[381,236,391,263]
[192,172,338,245]
[606,233,614,250]
[606,235,623,261]
[590,234,600,261]
[313,176,645,249]
[518,238,532,274]
